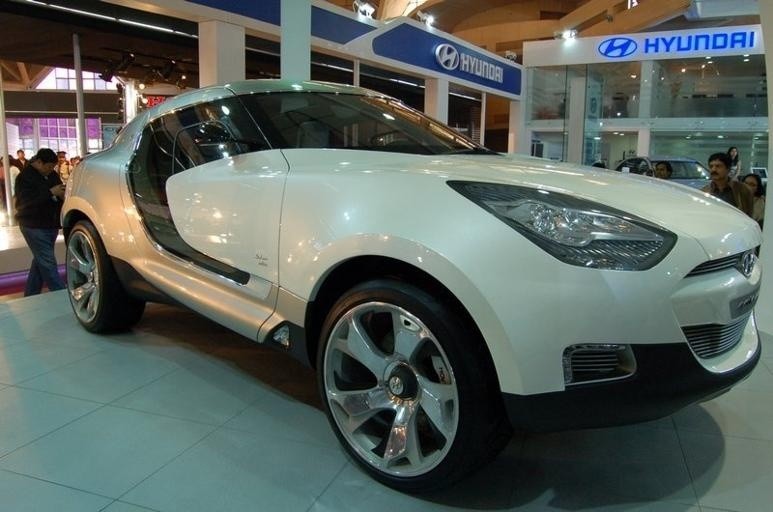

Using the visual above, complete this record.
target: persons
[15,147,66,297]
[654,161,673,180]
[740,172,765,257]
[699,151,754,221]
[42,151,81,230]
[0,149,28,226]
[727,146,741,182]
